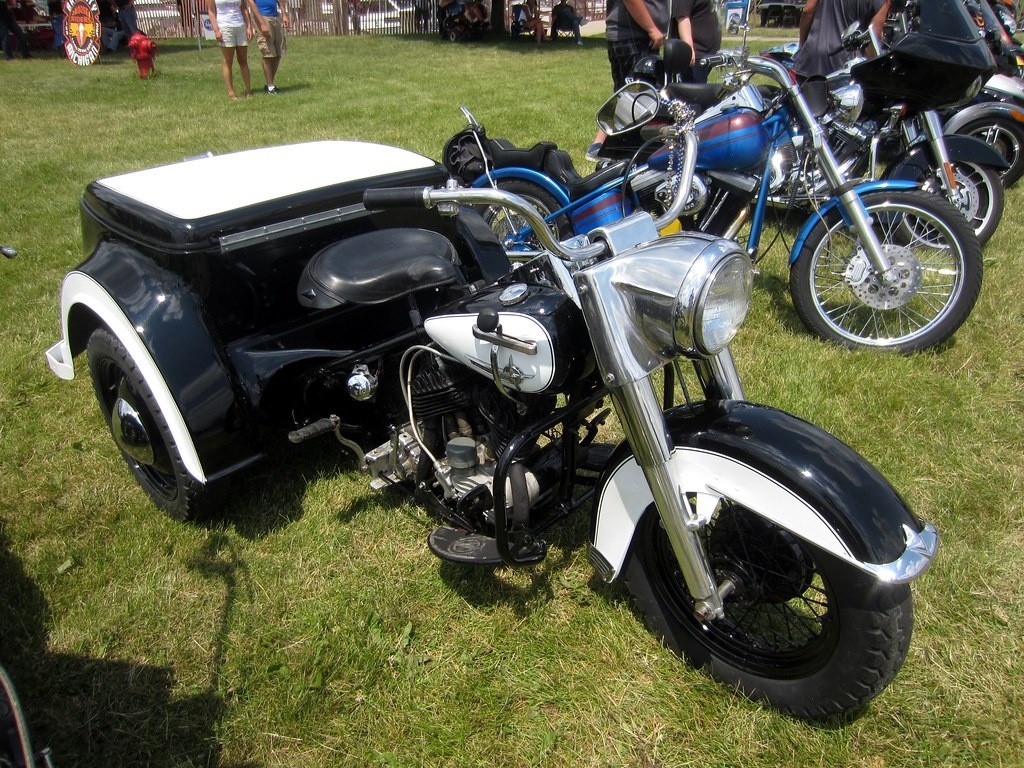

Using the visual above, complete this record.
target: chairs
[557,16,583,39]
[766,5,797,28]
[512,4,532,40]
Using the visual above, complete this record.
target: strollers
[442,0,488,42]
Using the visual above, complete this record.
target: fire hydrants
[128,31,156,80]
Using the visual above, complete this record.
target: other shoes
[545,36,552,41]
[577,41,583,45]
[264,85,281,96]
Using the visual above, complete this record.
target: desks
[758,3,806,26]
[13,24,66,59]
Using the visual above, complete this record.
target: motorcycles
[40,79,938,728]
[442,0,1024,359]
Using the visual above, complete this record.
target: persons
[670,0,721,85]
[412,0,584,47]
[794,0,892,107]
[207,0,253,99]
[604,1,671,99]
[248,0,291,96]
